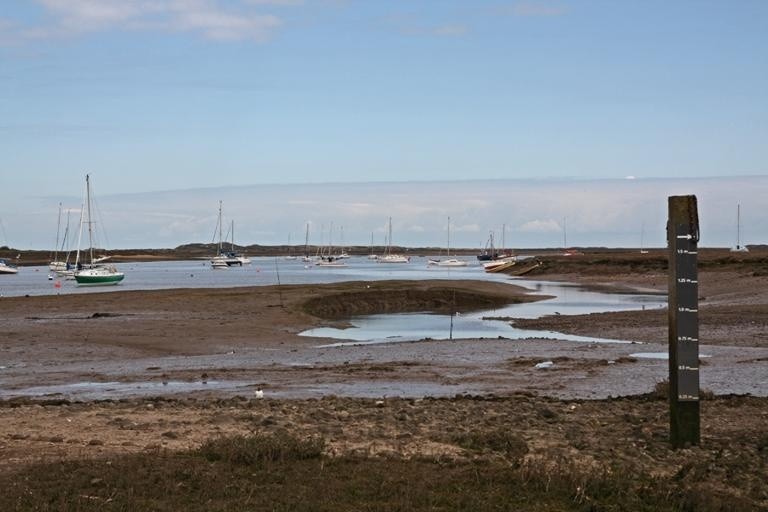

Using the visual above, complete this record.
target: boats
[0,258,16,273]
[483,261,543,275]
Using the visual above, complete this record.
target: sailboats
[285,221,350,267]
[49,174,124,284]
[477,223,515,262]
[428,217,466,267]
[209,201,250,267]
[368,218,409,263]
